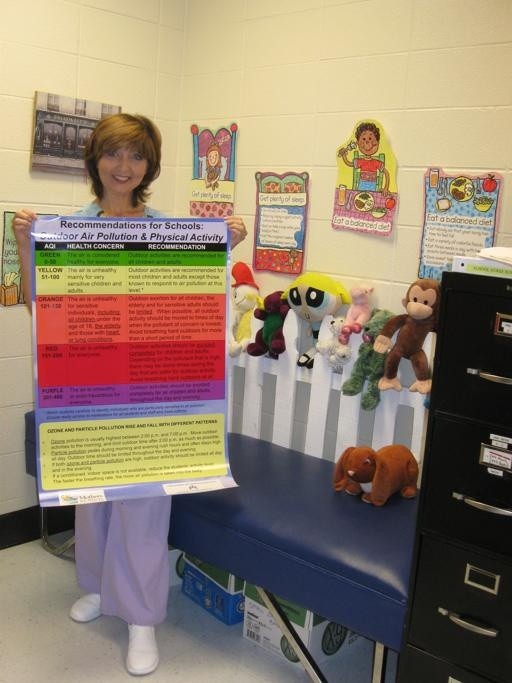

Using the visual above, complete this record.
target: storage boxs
[178,549,245,626]
[240,582,365,670]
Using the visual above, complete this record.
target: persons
[10,108,250,680]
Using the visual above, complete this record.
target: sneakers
[125,624,160,675]
[68,593,102,624]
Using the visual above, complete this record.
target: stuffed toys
[330,443,419,509]
[337,284,375,344]
[224,260,267,357]
[372,278,442,395]
[279,270,352,372]
[313,315,352,371]
[340,306,397,412]
[245,289,292,362]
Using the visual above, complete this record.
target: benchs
[25,408,421,683]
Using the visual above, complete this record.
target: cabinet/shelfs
[395,271,512,683]
